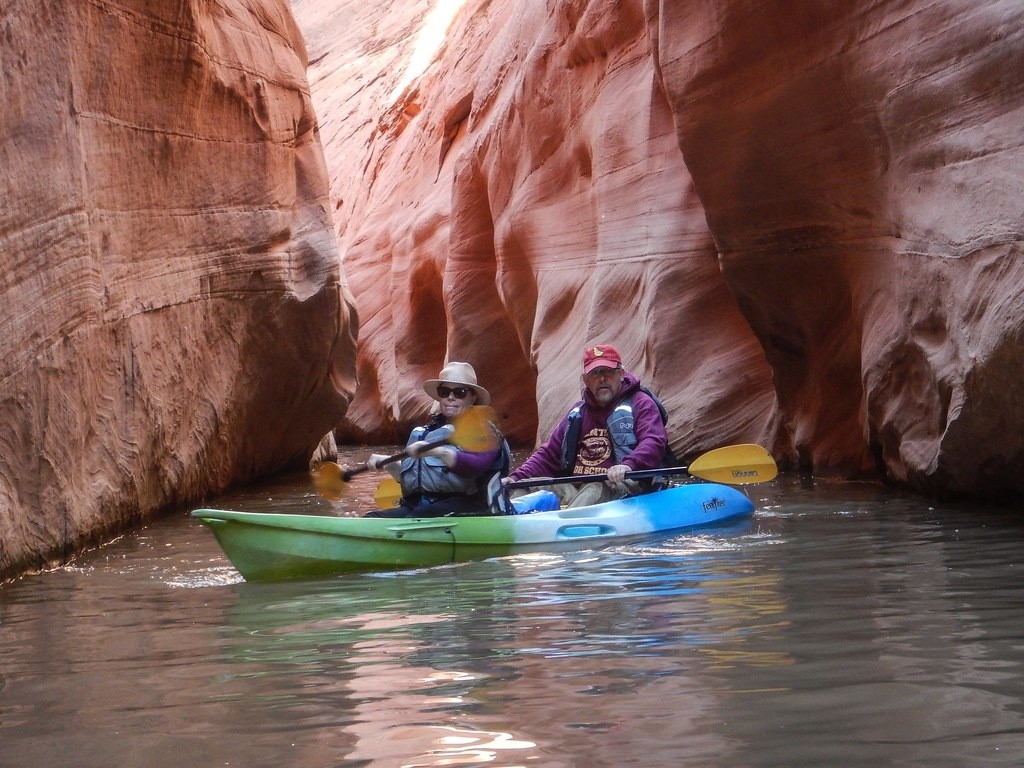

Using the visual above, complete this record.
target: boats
[191,481,756,585]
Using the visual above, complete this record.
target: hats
[583,344,622,374]
[424,362,491,406]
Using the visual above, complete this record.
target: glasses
[437,383,471,399]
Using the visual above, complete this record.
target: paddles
[311,403,503,499]
[372,442,781,511]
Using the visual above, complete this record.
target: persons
[361,360,510,518]
[484,345,669,516]
